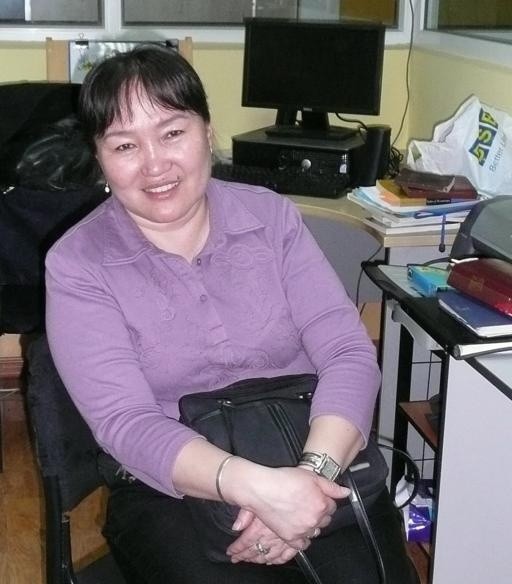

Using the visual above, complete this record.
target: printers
[448,194,512,265]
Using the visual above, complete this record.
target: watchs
[296,451,342,485]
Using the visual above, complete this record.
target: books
[400,253,512,360]
[349,180,486,233]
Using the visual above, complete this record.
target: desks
[352,253,510,584]
[284,186,485,494]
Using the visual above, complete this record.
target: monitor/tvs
[240,20,385,140]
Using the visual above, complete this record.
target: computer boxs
[232,125,369,186]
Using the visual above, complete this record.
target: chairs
[20,333,125,582]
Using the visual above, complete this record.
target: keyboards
[212,164,351,199]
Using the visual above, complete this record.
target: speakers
[362,124,392,186]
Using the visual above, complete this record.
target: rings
[307,528,321,539]
[256,544,268,554]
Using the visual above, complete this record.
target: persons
[44,42,415,584]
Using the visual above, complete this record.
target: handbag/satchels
[177,373,389,563]
